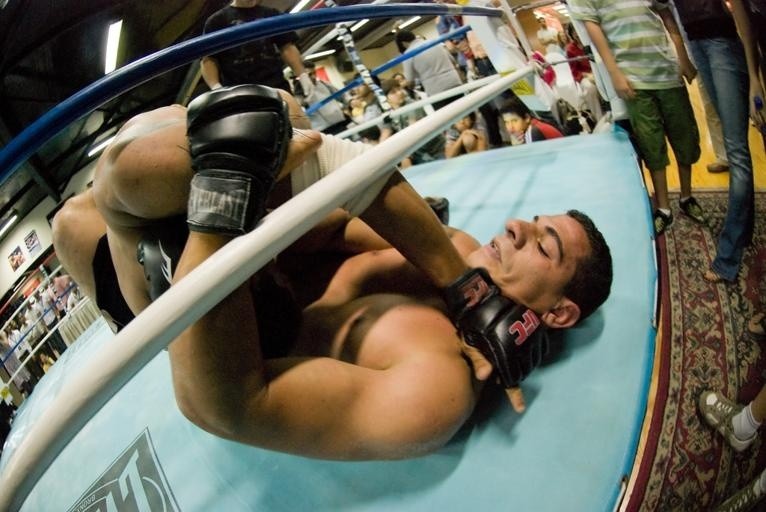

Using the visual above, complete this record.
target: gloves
[439,266,551,390]
[185,82,293,237]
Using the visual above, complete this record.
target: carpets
[616,187,765,510]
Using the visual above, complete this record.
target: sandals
[747,313,766,337]
[702,268,725,283]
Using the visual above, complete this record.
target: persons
[694,386,766,511]
[54,86,613,463]
[285,1,713,234]
[673,0,765,282]
[200,0,316,102]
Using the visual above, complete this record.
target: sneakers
[679,194,710,226]
[653,206,674,237]
[698,389,760,454]
[707,158,731,173]
[715,472,765,512]
